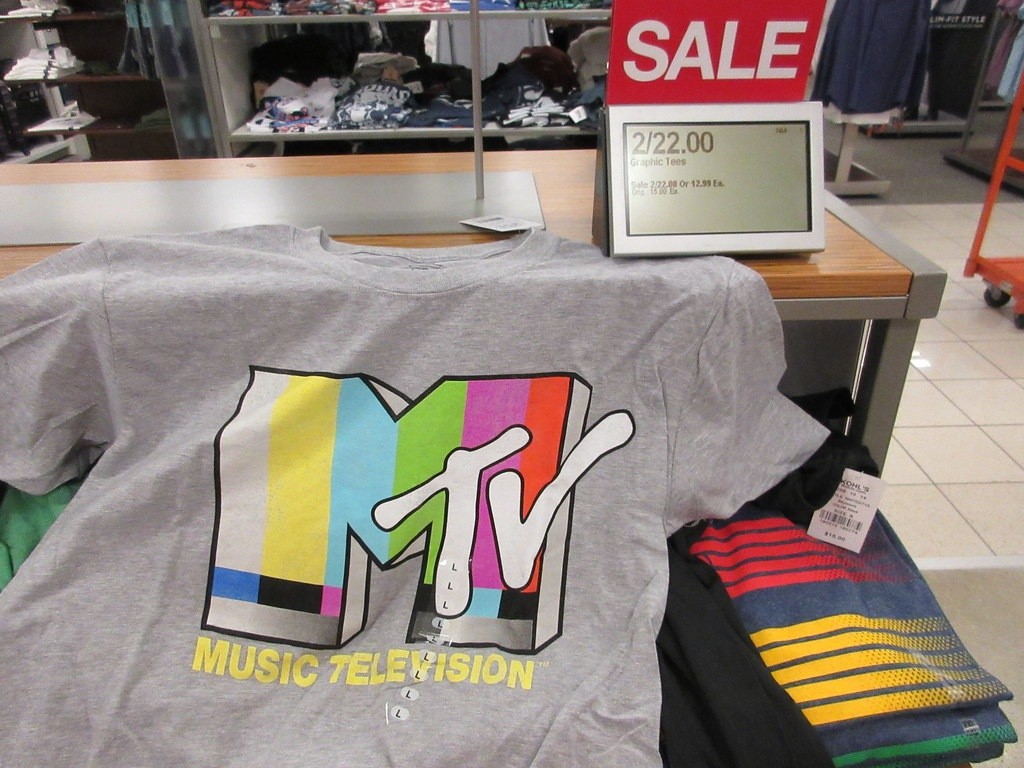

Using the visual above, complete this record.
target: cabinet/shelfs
[3,1,173,163]
[189,1,1024,199]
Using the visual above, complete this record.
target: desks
[1,148,949,482]
